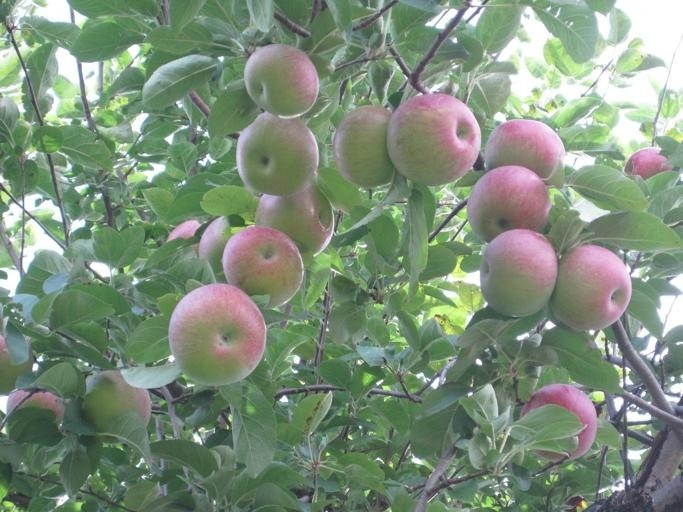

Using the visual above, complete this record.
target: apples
[0,335,151,432]
[519,384,597,461]
[167,42,632,382]
[625,147,673,180]
